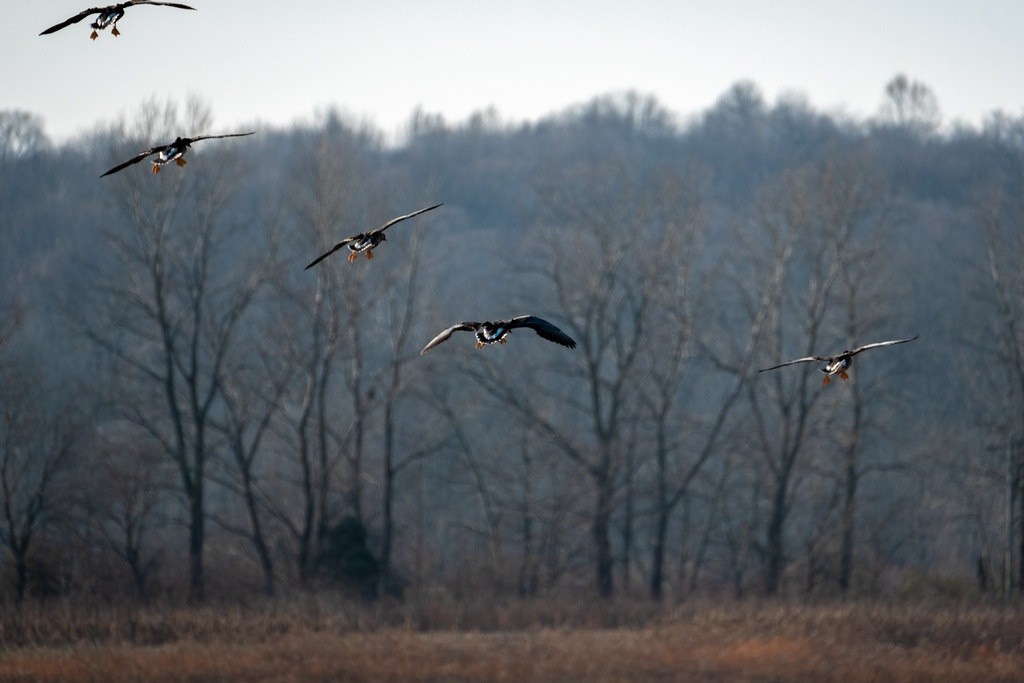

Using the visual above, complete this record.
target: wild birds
[39,0,198,41]
[304,203,446,270]
[759,334,920,386]
[99,131,256,179]
[420,315,577,356]
